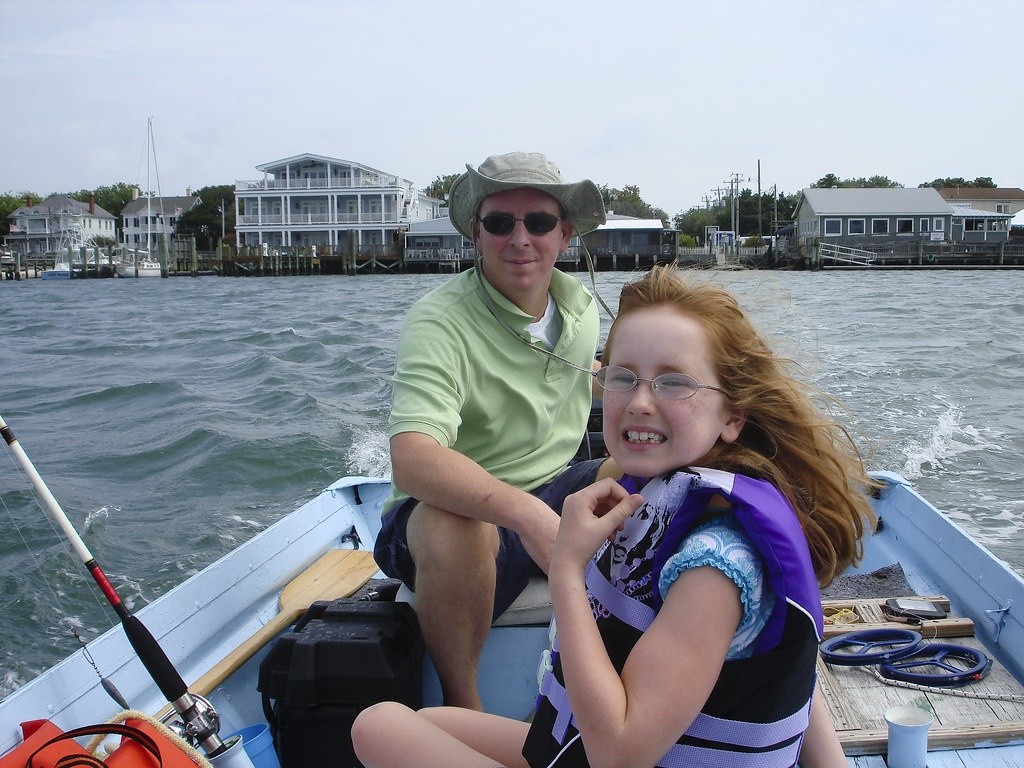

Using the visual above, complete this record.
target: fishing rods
[0,414,253,768]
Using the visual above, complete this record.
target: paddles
[151,548,381,724]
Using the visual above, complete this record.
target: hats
[448,151,606,244]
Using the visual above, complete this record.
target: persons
[373,151,624,716]
[350,260,886,767]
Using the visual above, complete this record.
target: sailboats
[111,117,170,278]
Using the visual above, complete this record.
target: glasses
[478,213,561,235]
[592,365,736,401]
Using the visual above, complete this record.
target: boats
[40,228,80,279]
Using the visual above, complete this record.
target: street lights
[747,177,762,247]
[768,187,778,236]
